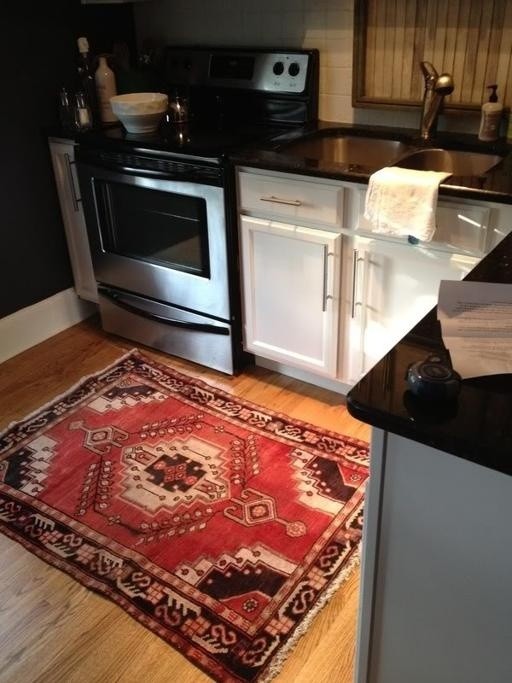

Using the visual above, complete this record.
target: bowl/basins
[108,92,168,134]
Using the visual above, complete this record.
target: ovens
[73,144,234,323]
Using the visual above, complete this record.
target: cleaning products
[478,86,504,142]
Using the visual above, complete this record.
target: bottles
[74,36,99,113]
[56,88,93,132]
[94,57,118,128]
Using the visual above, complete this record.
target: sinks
[390,148,504,178]
[280,135,409,168]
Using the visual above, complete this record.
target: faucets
[420,74,454,138]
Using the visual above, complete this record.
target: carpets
[1,346,371,683]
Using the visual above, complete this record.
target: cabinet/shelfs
[238,169,511,394]
